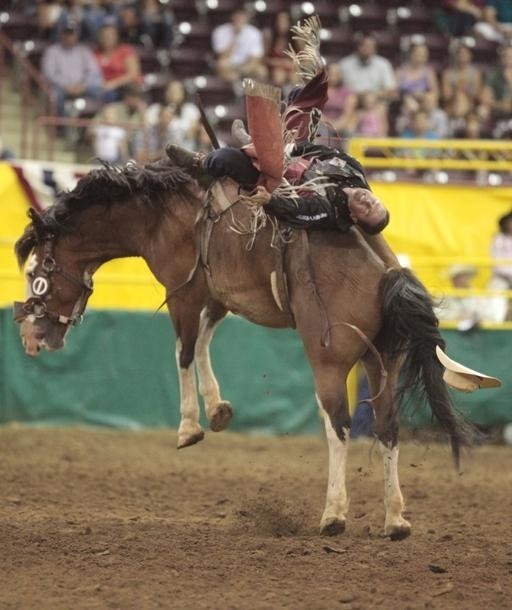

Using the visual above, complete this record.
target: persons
[428,262,492,322]
[483,209,511,322]
[8,0,511,186]
[165,13,392,237]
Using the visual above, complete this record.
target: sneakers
[228,118,252,151]
[165,143,200,168]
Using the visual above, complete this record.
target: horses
[12,152,488,542]
